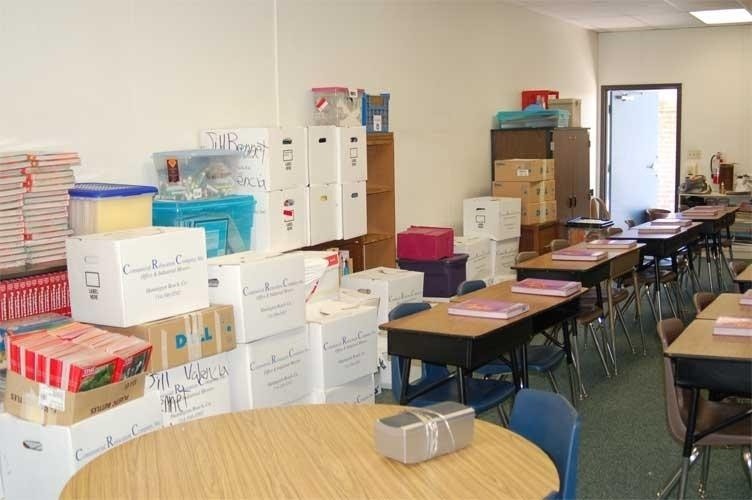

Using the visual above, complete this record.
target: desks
[510,240,647,401]
[609,221,703,323]
[661,293,750,499]
[60,404,561,500]
[665,205,744,298]
[378,279,590,408]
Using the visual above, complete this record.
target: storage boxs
[547,99,581,128]
[6,368,145,427]
[339,126,366,184]
[199,127,307,192]
[308,125,338,187]
[375,334,423,388]
[82,303,238,374]
[455,237,492,282]
[521,198,557,226]
[2,389,172,500]
[314,372,375,405]
[494,159,557,182]
[341,267,423,334]
[397,225,453,262]
[142,353,228,429]
[395,253,469,301]
[492,239,518,284]
[69,182,157,235]
[152,149,244,201]
[232,323,313,414]
[208,249,306,344]
[152,195,257,258]
[462,197,523,242]
[567,215,614,247]
[310,183,339,247]
[492,180,555,203]
[237,185,310,253]
[304,251,339,305]
[65,227,210,329]
[308,289,377,390]
[341,181,367,240]
[362,93,390,133]
[311,87,365,129]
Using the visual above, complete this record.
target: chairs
[652,317,752,500]
[693,230,736,286]
[693,291,721,314]
[388,305,516,429]
[555,303,611,400]
[508,387,583,499]
[579,283,636,377]
[727,257,745,287]
[515,252,617,379]
[656,251,697,317]
[458,280,565,394]
[619,266,677,336]
[550,239,637,367]
[559,251,561,254]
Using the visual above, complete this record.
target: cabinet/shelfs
[490,126,591,257]
[317,131,398,273]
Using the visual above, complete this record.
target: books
[551,247,608,262]
[0,149,82,276]
[511,277,582,296]
[694,205,727,210]
[651,218,692,227]
[638,225,681,233]
[587,240,637,249]
[448,296,531,319]
[5,321,152,393]
[713,316,752,336]
[734,201,752,224]
[682,209,718,216]
[738,288,752,309]
[0,270,70,322]
